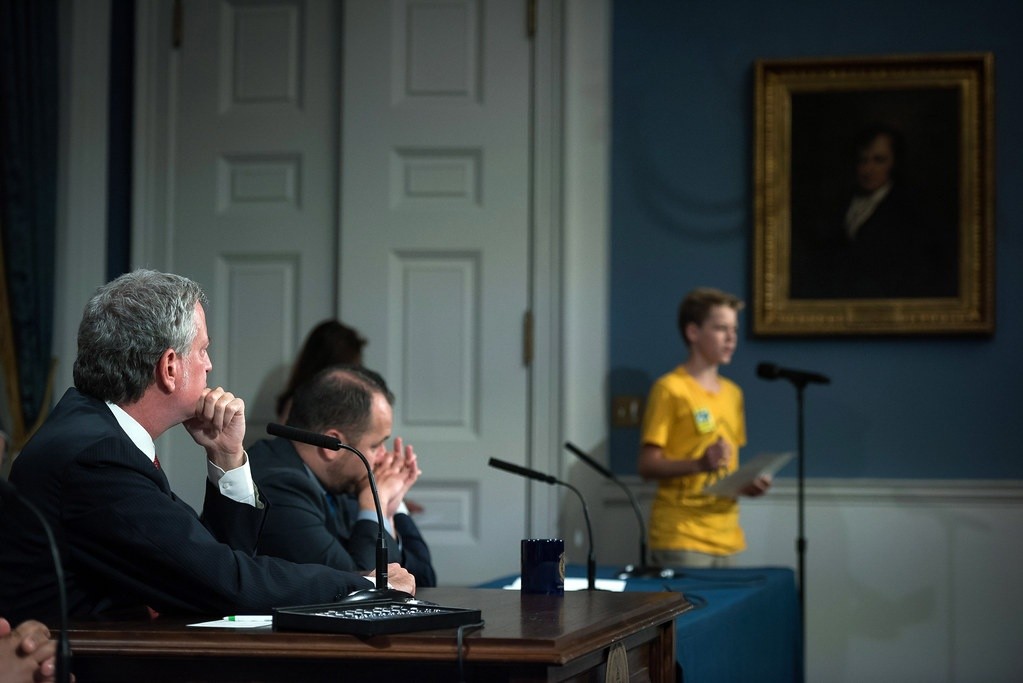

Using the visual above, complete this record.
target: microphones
[487,456,609,592]
[262,421,414,602]
[564,442,672,580]
[754,359,832,387]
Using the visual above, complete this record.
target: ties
[154,454,166,477]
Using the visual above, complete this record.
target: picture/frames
[753,50,992,334]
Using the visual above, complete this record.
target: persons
[801,123,931,299]
[0,270,415,683]
[0,617,57,683]
[636,288,773,568]
[247,317,437,589]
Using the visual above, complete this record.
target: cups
[520,539,566,598]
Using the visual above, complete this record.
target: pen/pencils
[223,615,272,622]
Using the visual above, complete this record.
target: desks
[473,563,800,683]
[45,585,695,683]
